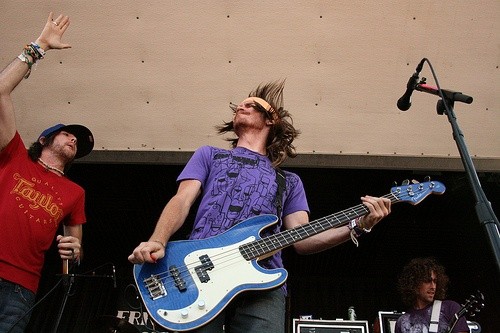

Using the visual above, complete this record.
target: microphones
[59,240,69,282]
[397,58,426,111]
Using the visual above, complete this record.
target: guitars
[134,178,444,331]
[446,291,486,332]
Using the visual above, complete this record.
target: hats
[37,124,94,158]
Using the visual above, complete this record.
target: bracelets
[152,240,165,249]
[17,42,46,79]
[348,216,372,246]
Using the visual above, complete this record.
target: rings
[72,255,74,260]
[52,20,57,26]
[72,248,74,255]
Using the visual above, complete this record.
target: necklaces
[39,158,65,176]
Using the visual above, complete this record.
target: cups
[348,307,355,320]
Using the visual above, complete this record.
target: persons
[0,11,94,333]
[395,258,470,333]
[128,83,392,333]
